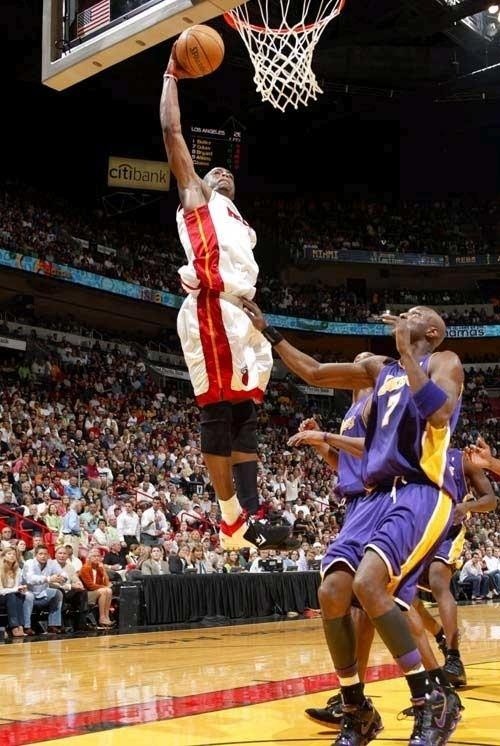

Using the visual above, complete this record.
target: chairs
[461,397,500,421]
[0,605,115,638]
[454,570,472,600]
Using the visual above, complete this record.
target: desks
[115,571,321,627]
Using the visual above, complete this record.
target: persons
[1,190,499,635]
[285,349,462,727]
[156,37,304,553]
[238,294,466,746]
[410,442,497,688]
[462,437,500,477]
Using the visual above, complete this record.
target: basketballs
[175,25,225,75]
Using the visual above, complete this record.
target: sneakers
[218,507,302,551]
[306,687,385,746]
[396,633,464,746]
